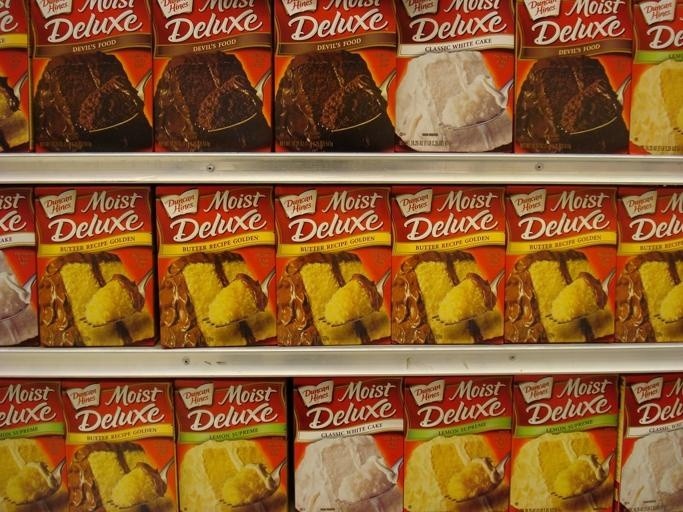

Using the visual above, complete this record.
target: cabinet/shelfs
[0,153,681,378]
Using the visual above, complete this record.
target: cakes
[0,73,29,152]
[504,250,615,344]
[0,250,39,347]
[510,433,613,512]
[404,436,509,511]
[274,52,395,152]
[33,52,153,152]
[159,251,277,347]
[68,441,174,512]
[392,251,504,345]
[621,429,683,511]
[179,440,287,512]
[0,438,69,512]
[630,60,683,154]
[516,55,630,155]
[39,251,154,348]
[153,52,273,153]
[615,251,683,343]
[395,51,515,154]
[295,435,403,512]
[275,249,391,346]
